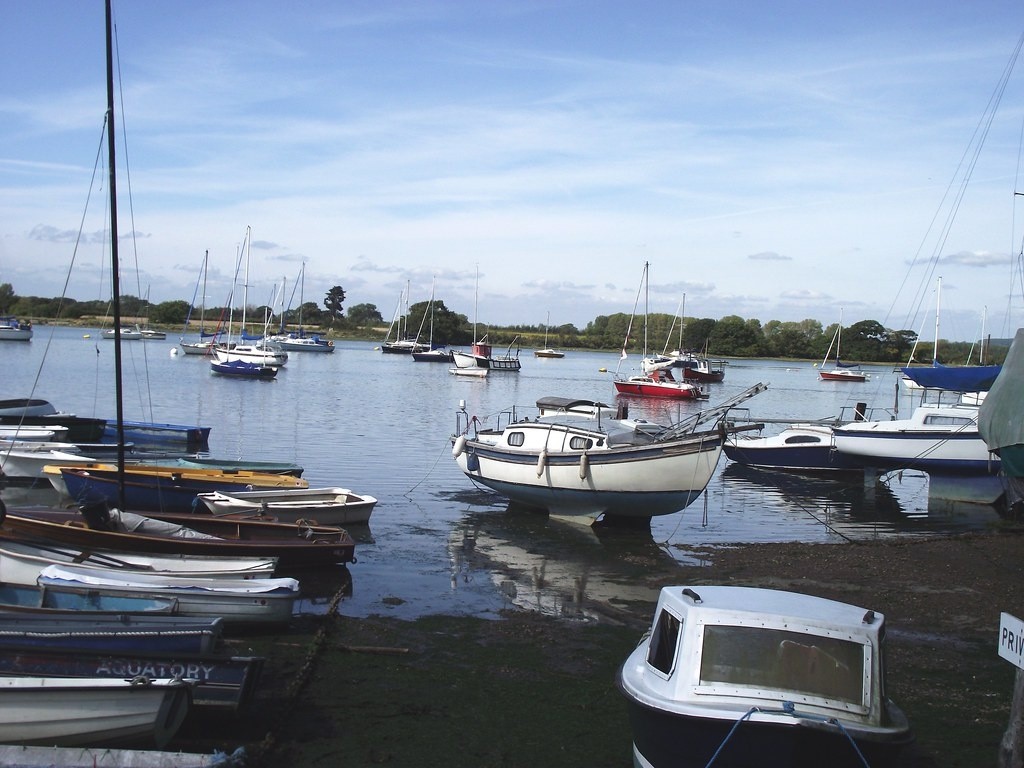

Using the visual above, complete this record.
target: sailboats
[960,306,990,406]
[900,276,950,392]
[819,307,866,381]
[381,281,430,355]
[98,268,142,341]
[642,292,728,382]
[132,284,167,340]
[613,261,711,399]
[411,275,455,364]
[452,264,522,372]
[179,225,335,378]
[534,311,565,358]
[0,1,358,569]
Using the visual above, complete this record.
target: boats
[449,366,490,377]
[0,315,33,341]
[450,381,768,515]
[0,399,375,745]
[723,365,1001,476]
[615,587,916,768]
